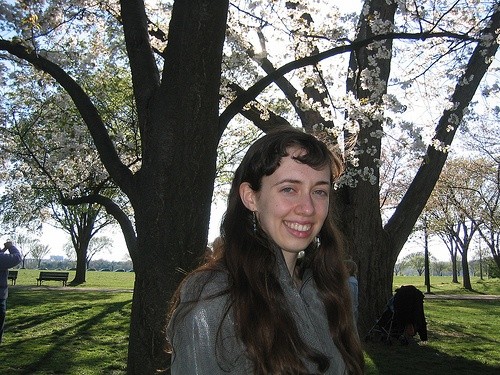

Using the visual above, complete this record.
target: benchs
[35,271,69,287]
[8,270,18,286]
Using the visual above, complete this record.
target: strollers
[364,291,410,346]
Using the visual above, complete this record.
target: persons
[0,241,22,345]
[203,236,225,264]
[155,127,367,375]
[342,260,359,324]
